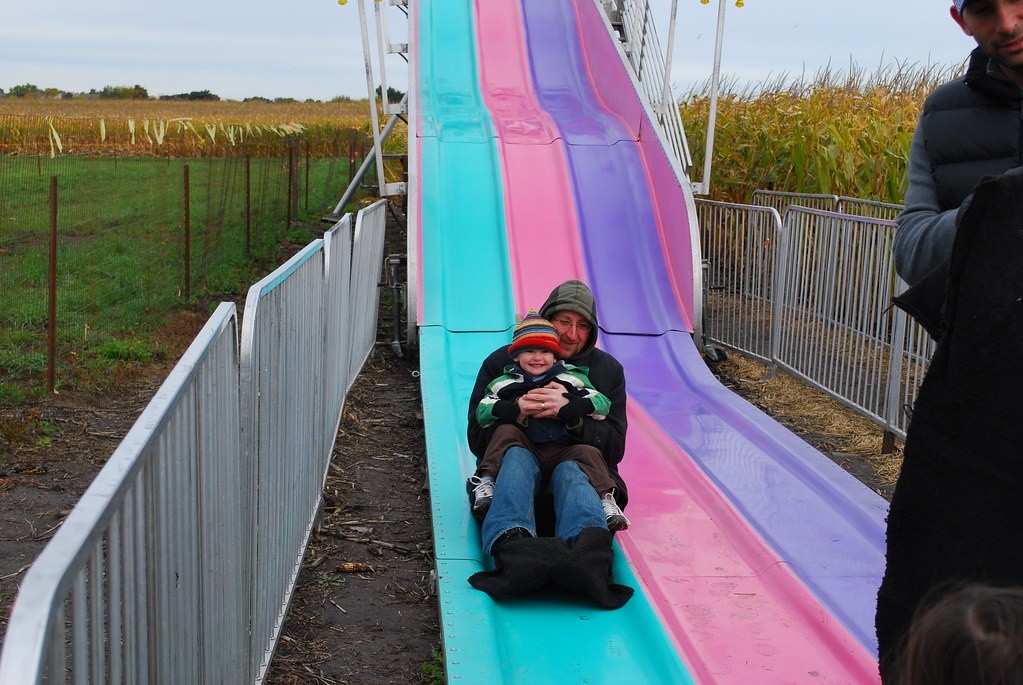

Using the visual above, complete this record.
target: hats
[507,307,564,360]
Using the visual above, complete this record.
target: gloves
[557,393,595,423]
[491,397,521,422]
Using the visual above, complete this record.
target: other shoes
[490,527,531,555]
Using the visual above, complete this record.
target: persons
[466,280,635,611]
[469,308,631,531]
[891,1,1023,287]
[895,584,1023,685]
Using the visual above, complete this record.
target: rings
[541,403,545,410]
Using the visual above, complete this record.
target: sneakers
[470,476,495,510]
[600,488,631,531]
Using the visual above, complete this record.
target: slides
[403,2,895,685]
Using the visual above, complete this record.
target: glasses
[551,314,594,335]
